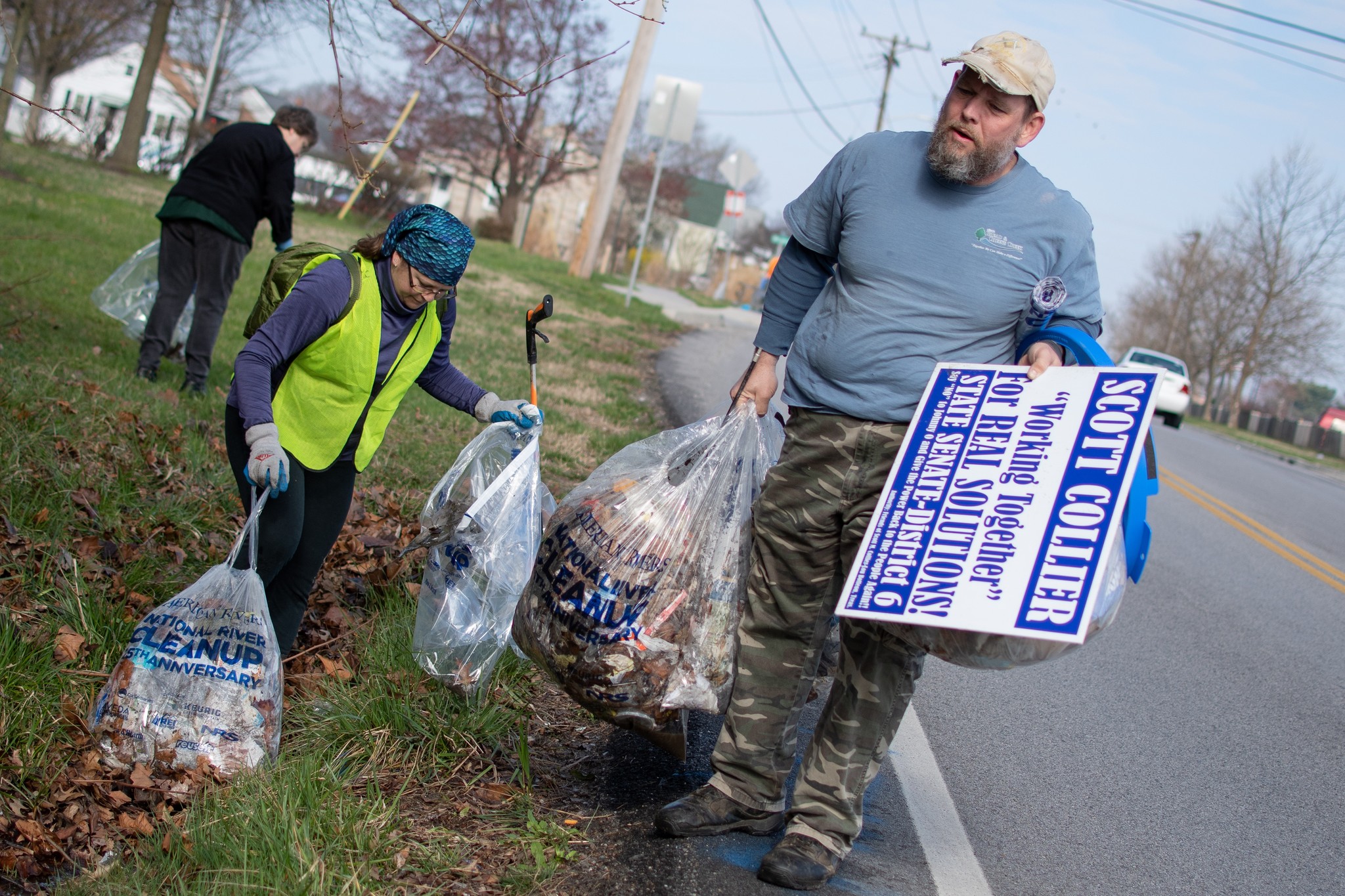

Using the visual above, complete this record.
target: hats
[943,31,1055,112]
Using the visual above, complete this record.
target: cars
[1114,346,1190,430]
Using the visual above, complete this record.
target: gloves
[245,422,290,498]
[473,391,544,433]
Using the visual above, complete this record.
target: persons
[651,31,1103,892]
[224,203,543,661]
[134,104,318,401]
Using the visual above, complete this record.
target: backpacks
[242,241,447,349]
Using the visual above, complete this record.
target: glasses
[408,264,458,299]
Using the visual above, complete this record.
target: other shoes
[179,381,207,399]
[137,367,158,382]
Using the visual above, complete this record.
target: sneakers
[759,833,841,888]
[653,782,784,835]
